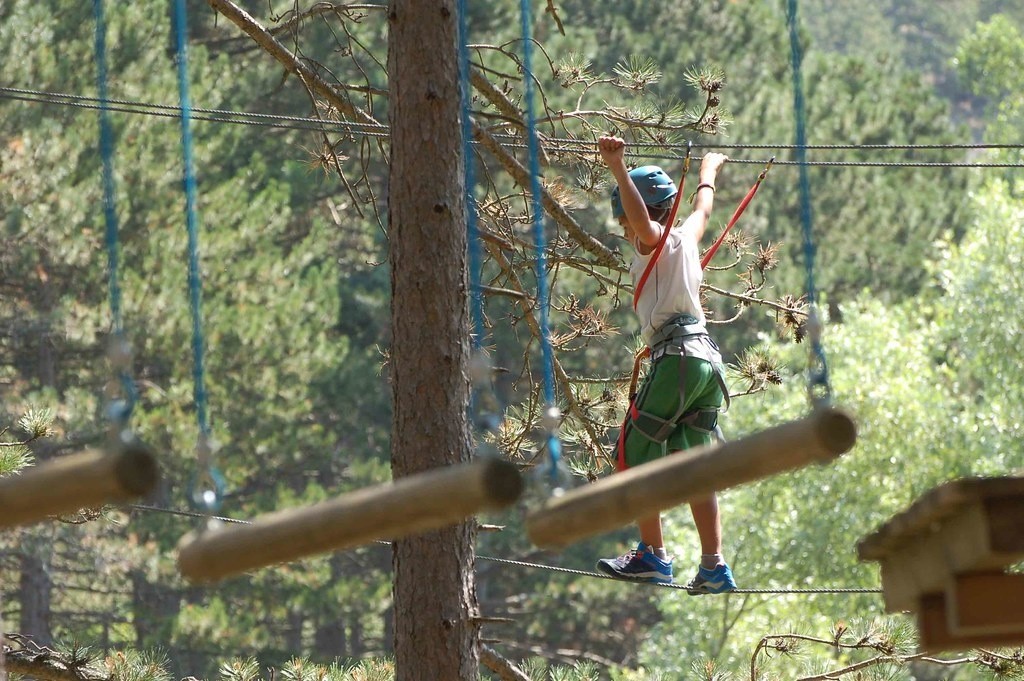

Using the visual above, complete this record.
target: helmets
[611,165,679,218]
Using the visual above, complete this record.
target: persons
[598,134,738,595]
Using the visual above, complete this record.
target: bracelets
[696,183,716,192]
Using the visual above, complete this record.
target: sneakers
[596,541,673,583]
[687,563,737,596]
